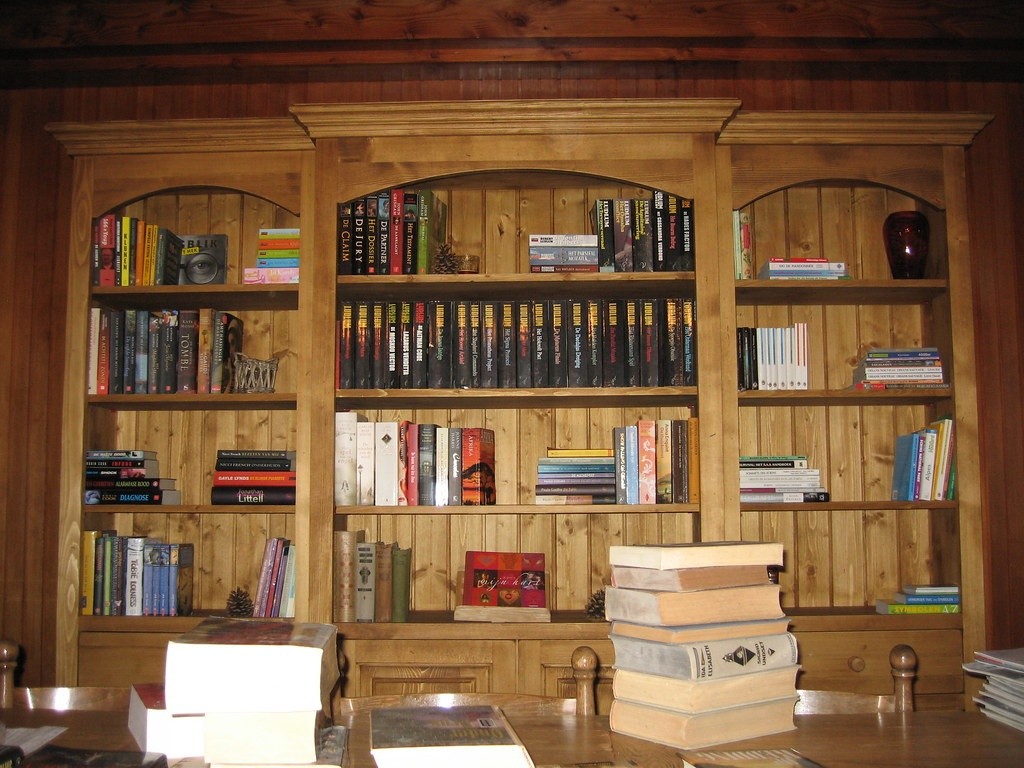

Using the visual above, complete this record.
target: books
[82,190,1024,766]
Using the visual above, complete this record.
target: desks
[0,705,1023,768]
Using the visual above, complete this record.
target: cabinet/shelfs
[45,96,994,705]
